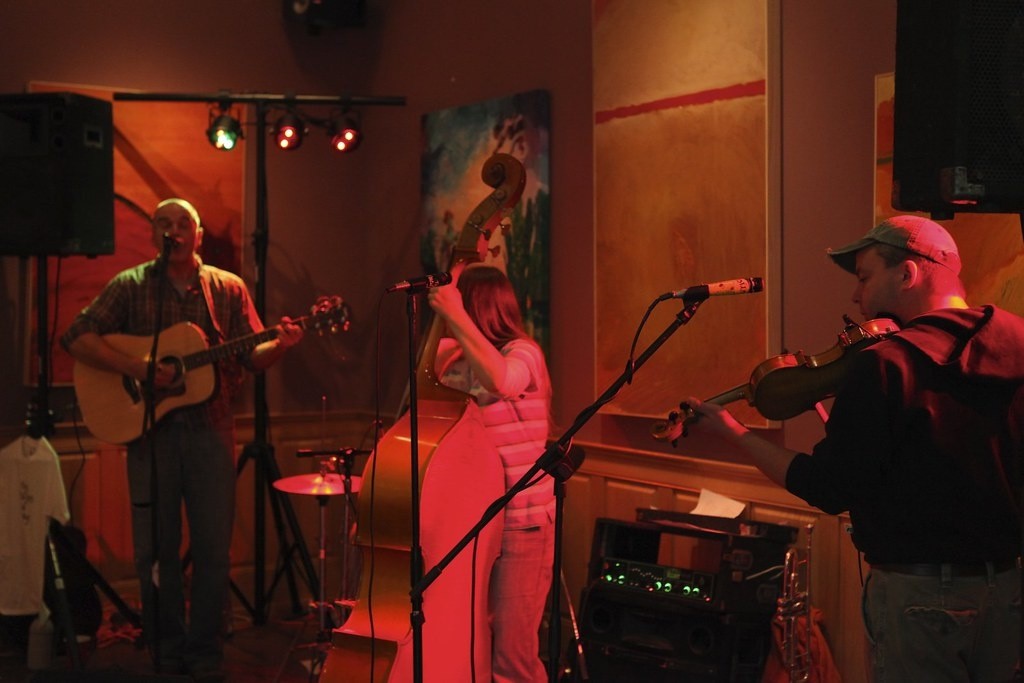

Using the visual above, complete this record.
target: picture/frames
[418,88,550,369]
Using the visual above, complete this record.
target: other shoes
[154,660,188,681]
[191,655,227,682]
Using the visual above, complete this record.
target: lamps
[268,93,309,151]
[206,90,244,151]
[319,95,363,153]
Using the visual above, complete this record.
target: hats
[828,215,964,276]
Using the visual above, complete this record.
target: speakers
[891,0,1024,220]
[1,92,115,256]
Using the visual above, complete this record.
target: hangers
[0,415,60,464]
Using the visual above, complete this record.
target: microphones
[164,233,184,249]
[674,277,764,298]
[394,272,452,293]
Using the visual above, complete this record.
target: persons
[416,262,556,683]
[59,197,304,683]
[677,215,1024,683]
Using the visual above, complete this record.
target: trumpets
[774,521,817,683]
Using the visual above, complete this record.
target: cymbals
[271,472,361,496]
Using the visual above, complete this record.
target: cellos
[317,151,527,682]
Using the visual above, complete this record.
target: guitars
[71,295,352,445]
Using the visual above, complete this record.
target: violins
[651,312,902,449]
